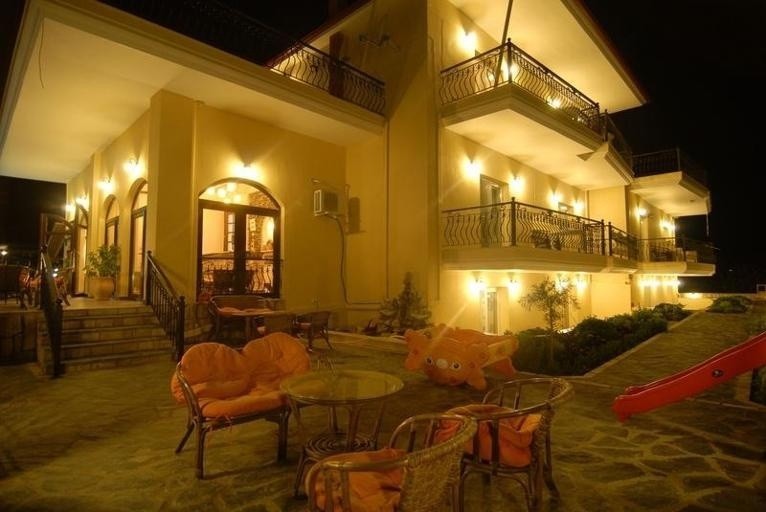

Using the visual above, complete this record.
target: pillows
[242,331,312,393]
[171,344,251,403]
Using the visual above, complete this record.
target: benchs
[208,295,274,342]
[175,349,334,479]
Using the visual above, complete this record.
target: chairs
[434,376,575,512]
[294,311,332,348]
[305,414,478,512]
[0,264,31,311]
[30,269,70,307]
[251,312,296,340]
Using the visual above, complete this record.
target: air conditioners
[314,190,345,216]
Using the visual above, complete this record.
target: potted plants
[83,244,122,301]
